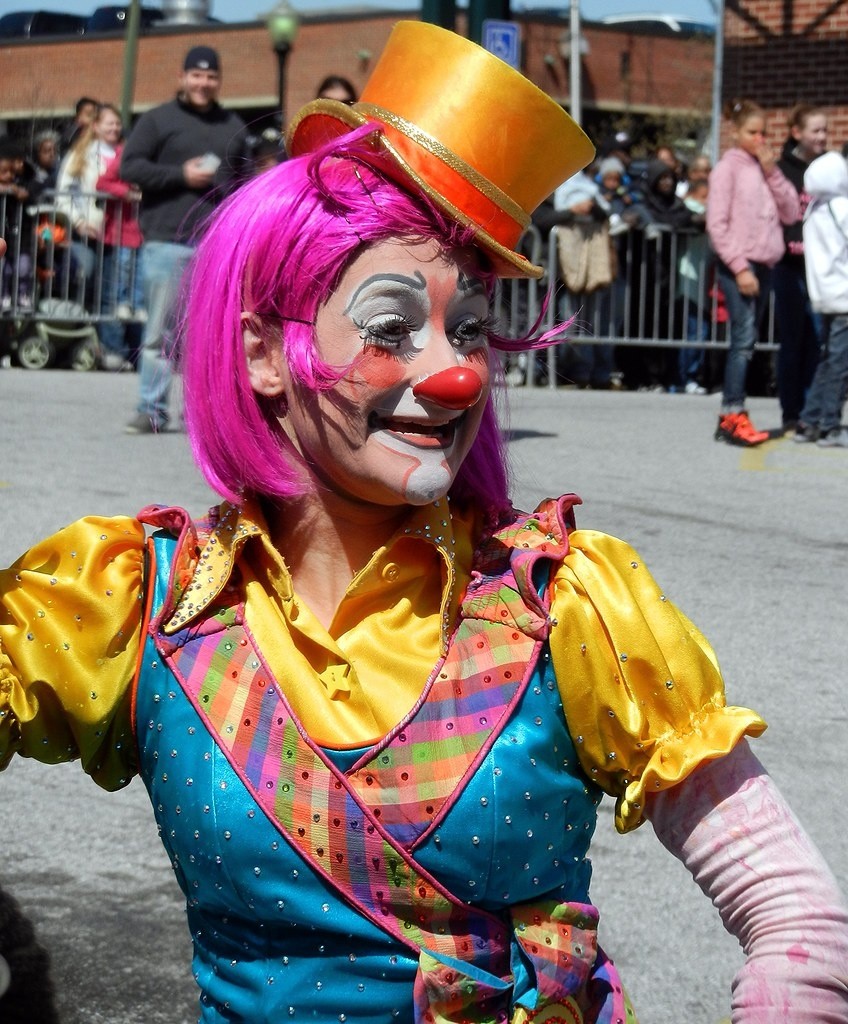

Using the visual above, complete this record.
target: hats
[284,18,596,277]
[184,45,220,71]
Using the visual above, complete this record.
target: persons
[0,15,848,1024]
[1,73,847,451]
[116,44,253,437]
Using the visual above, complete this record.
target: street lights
[267,4,296,142]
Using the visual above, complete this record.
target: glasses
[321,95,354,109]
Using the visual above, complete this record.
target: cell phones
[195,152,221,172]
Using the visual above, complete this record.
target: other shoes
[124,412,156,436]
[808,423,848,448]
[100,352,125,370]
[494,351,706,394]
[794,416,813,435]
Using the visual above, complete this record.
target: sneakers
[711,410,770,445]
